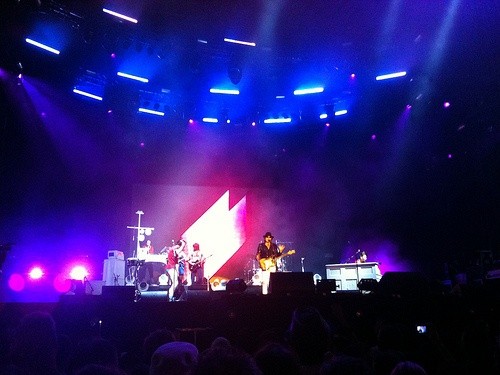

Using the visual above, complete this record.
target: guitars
[260,250,296,271]
[189,253,214,271]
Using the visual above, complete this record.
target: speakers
[268,272,315,295]
[140,290,212,302]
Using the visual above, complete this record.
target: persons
[140,240,156,284]
[0,301,500,375]
[356,248,367,263]
[164,239,206,302]
[113,273,120,286]
[254,231,285,296]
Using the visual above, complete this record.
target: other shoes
[175,297,184,302]
[167,298,173,302]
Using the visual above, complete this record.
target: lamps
[334,103,347,116]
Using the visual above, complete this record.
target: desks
[325,262,380,289]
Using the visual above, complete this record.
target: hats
[150,341,200,375]
[283,306,330,345]
[263,232,274,238]
